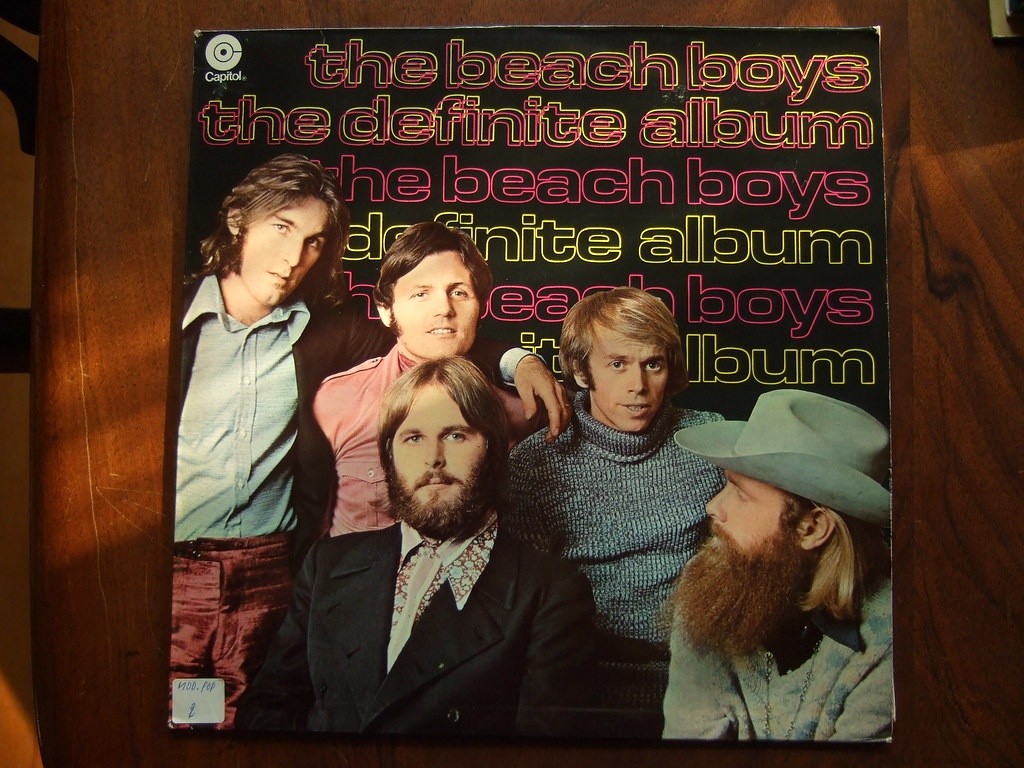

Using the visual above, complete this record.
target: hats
[673,389,891,525]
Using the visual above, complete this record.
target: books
[168,25,896,742]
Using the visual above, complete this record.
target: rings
[565,404,569,406]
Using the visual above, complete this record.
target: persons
[312,221,541,537]
[235,357,602,739]
[168,154,573,729]
[506,286,728,739]
[662,390,893,741]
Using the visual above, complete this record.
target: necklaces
[764,639,821,739]
